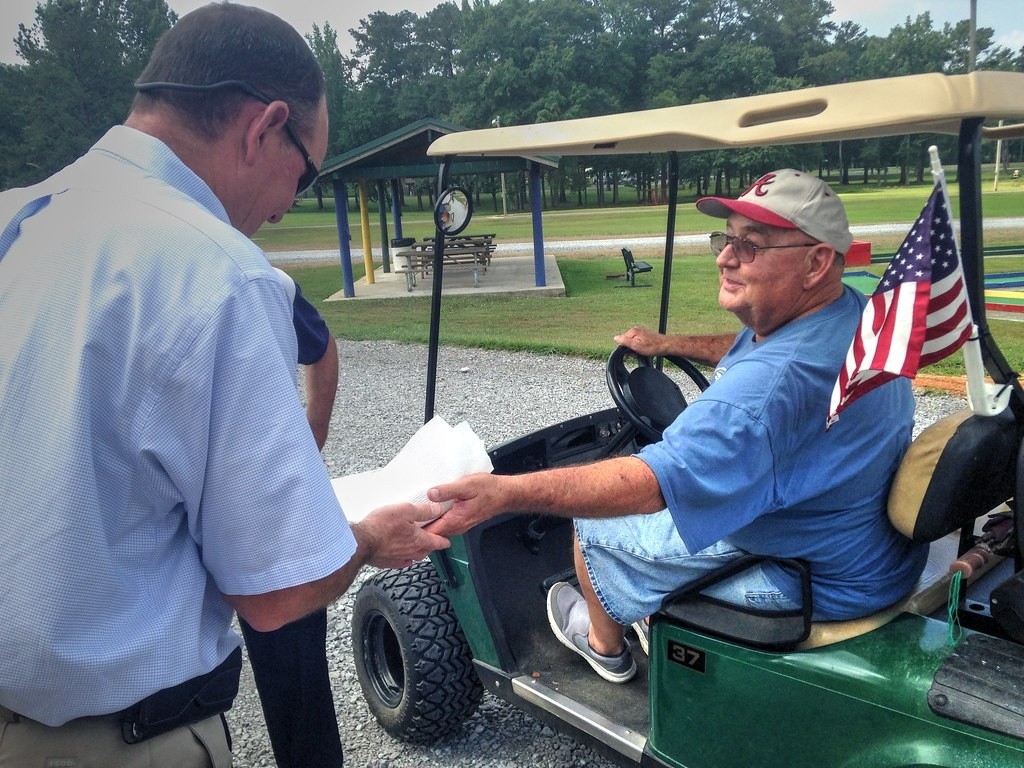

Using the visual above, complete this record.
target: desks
[422,233,496,275]
[411,238,492,279]
[396,247,485,292]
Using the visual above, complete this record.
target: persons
[218,281,343,768]
[438,202,448,222]
[449,191,456,204]
[423,168,929,683]
[0,2,452,768]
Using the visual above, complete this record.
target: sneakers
[546,582,638,683]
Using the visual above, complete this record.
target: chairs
[732,396,1023,651]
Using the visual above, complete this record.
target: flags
[826,181,973,432]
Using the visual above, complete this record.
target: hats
[695,168,853,256]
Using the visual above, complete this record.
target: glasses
[710,228,819,263]
[247,87,319,197]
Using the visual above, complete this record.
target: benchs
[622,247,654,286]
[396,244,497,291]
